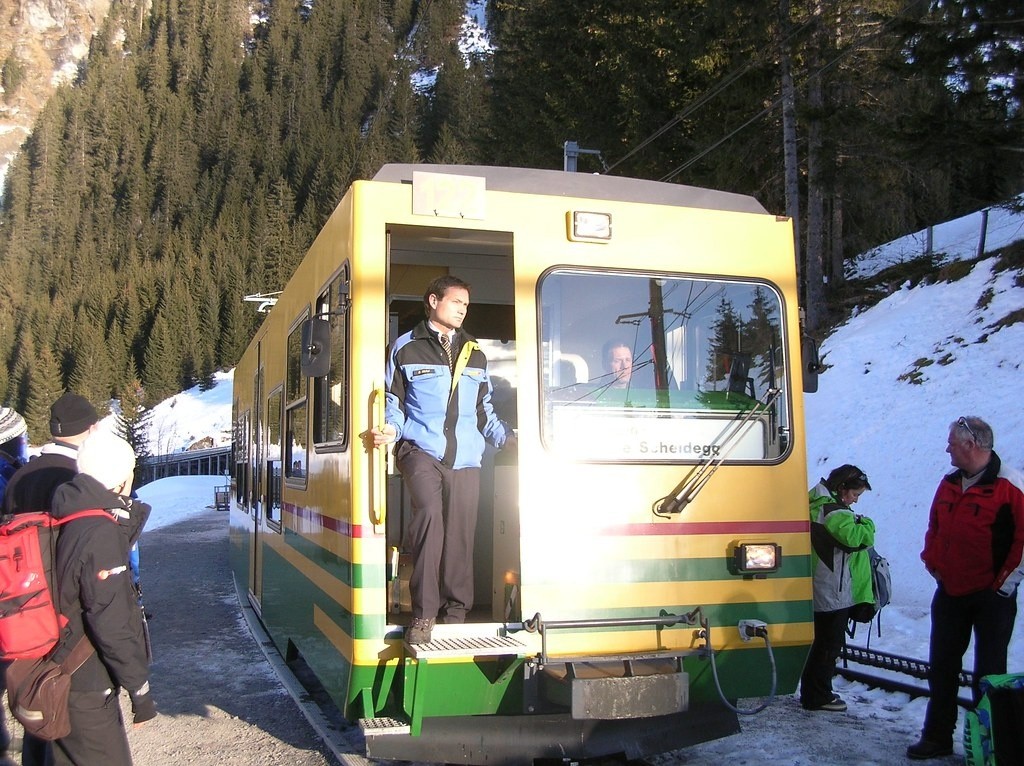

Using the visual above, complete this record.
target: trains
[228,139,818,766]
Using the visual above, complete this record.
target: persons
[292,461,305,478]
[799,465,892,712]
[0,405,29,515]
[0,392,142,601]
[600,338,639,388]
[372,276,519,642]
[906,416,1024,765]
[0,431,159,766]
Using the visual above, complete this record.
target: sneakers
[405,614,436,643]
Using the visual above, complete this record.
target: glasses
[957,417,977,442]
[844,474,868,484]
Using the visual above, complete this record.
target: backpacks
[849,548,892,623]
[964,675,1024,766]
[0,508,115,660]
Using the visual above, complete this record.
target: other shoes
[907,731,955,759]
[823,693,847,710]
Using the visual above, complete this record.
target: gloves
[129,690,157,723]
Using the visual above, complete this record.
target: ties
[440,333,453,375]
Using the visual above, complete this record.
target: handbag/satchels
[4,655,71,740]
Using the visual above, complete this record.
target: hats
[0,404,27,444]
[75,431,136,490]
[49,394,98,436]
